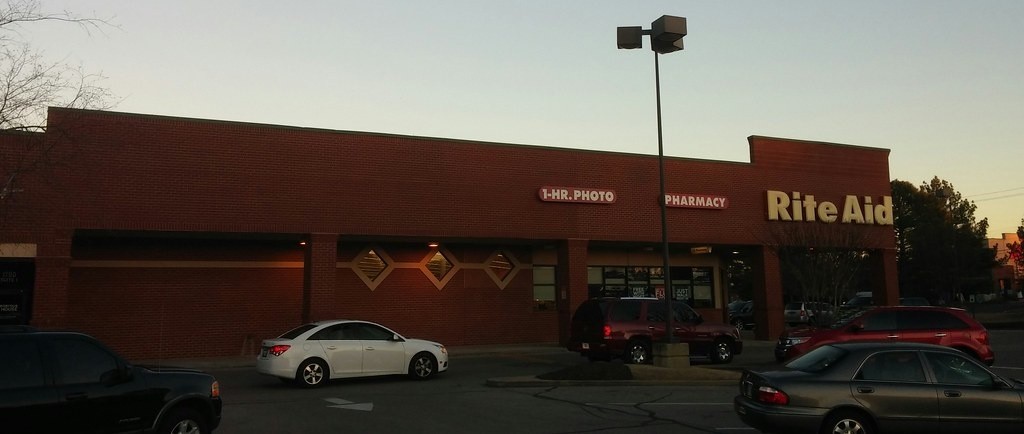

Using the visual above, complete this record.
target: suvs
[781,306,996,372]
[568,297,742,366]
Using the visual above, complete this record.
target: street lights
[615,14,689,338]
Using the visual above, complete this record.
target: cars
[255,318,449,389]
[729,297,756,326]
[0,329,222,434]
[734,339,1024,434]
[785,299,836,328]
